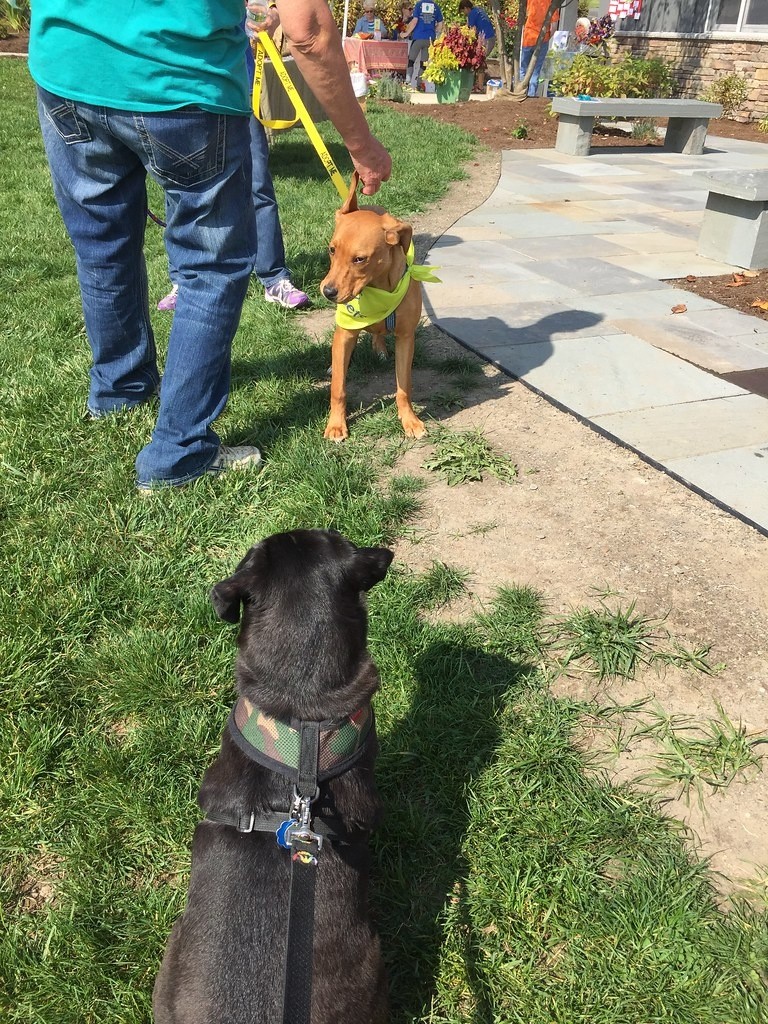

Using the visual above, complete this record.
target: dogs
[151,528,396,1024]
[319,169,428,442]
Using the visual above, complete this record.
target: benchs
[552,96,722,156]
[694,168,768,270]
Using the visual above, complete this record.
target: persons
[520,0,559,98]
[459,0,497,94]
[394,2,419,86]
[353,0,389,41]
[400,0,443,93]
[19,0,395,497]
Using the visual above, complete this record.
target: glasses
[366,10,374,13]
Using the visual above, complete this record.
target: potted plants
[421,20,489,104]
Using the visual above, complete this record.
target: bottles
[245,0,269,40]
[374,29,381,41]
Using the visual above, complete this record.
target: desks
[344,36,408,84]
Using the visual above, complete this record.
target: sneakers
[140,444,260,495]
[157,283,178,310]
[264,277,309,308]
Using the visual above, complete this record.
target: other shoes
[397,76,411,83]
[470,86,485,93]
